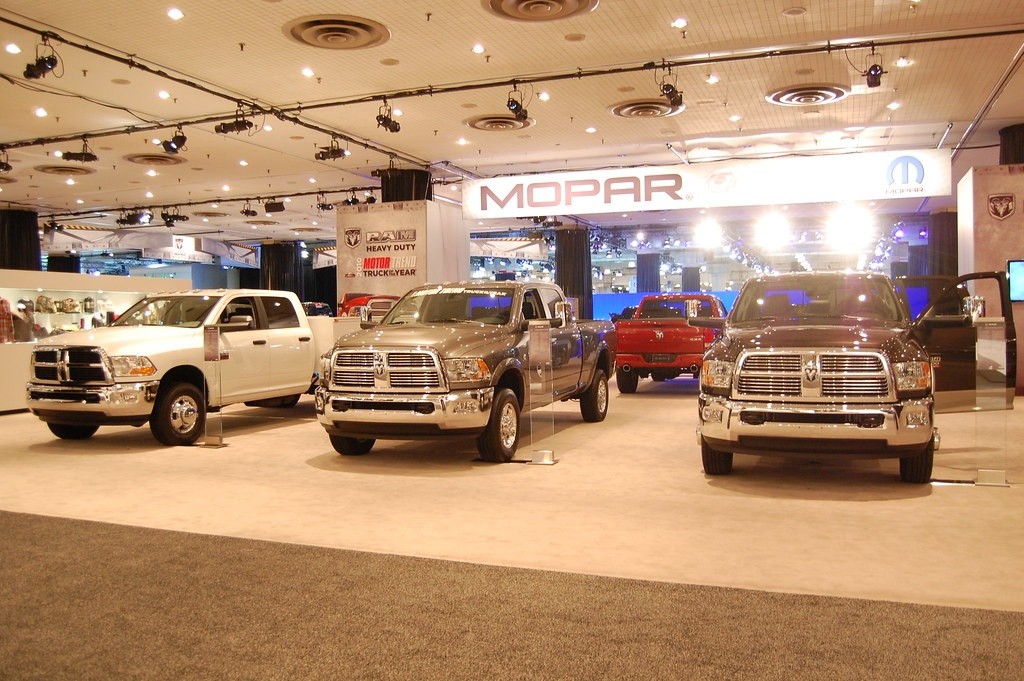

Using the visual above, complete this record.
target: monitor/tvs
[1006,260,1024,302]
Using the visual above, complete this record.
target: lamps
[215,102,253,134]
[315,195,333,210]
[865,44,885,88]
[265,198,286,212]
[161,213,188,228]
[371,155,397,178]
[162,126,188,156]
[377,97,401,132]
[22,34,65,80]
[0,148,13,172]
[62,138,97,163]
[101,251,114,257]
[655,65,683,108]
[342,189,374,206]
[507,82,528,122]
[531,216,563,228]
[117,210,153,226]
[43,220,66,234]
[315,139,346,161]
[239,202,258,216]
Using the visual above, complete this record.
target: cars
[301,302,334,318]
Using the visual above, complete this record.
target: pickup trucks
[314,282,612,464]
[698,272,1017,485]
[27,288,363,448]
[614,295,728,395]
[338,292,419,318]
